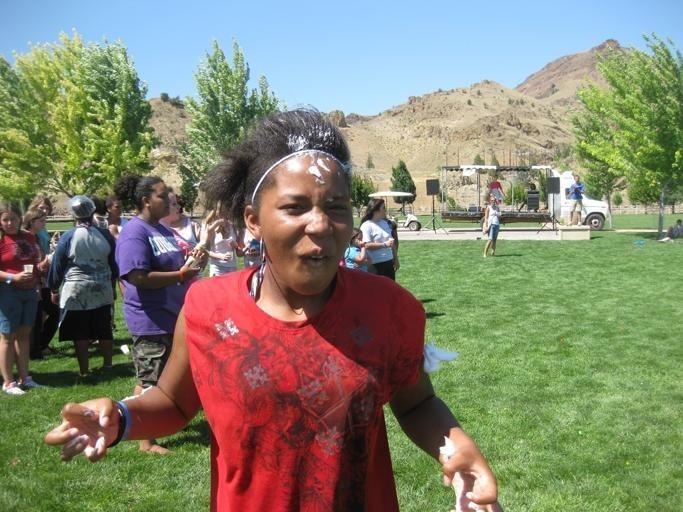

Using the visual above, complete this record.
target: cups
[23,264,34,274]
[119,344,130,355]
[185,256,198,267]
[389,238,395,245]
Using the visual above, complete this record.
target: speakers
[426,179,439,195]
[527,194,539,212]
[546,177,560,194]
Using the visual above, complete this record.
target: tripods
[417,195,449,235]
[536,194,562,234]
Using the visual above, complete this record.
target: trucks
[440,164,608,232]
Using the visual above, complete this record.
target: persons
[44,106,506,512]
[489,175,505,205]
[518,184,536,212]
[666,219,683,238]
[567,175,586,227]
[482,194,501,258]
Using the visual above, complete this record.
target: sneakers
[148,444,169,456]
[78,370,92,378]
[16,375,42,389]
[1,380,26,396]
[30,347,59,361]
[95,366,112,373]
